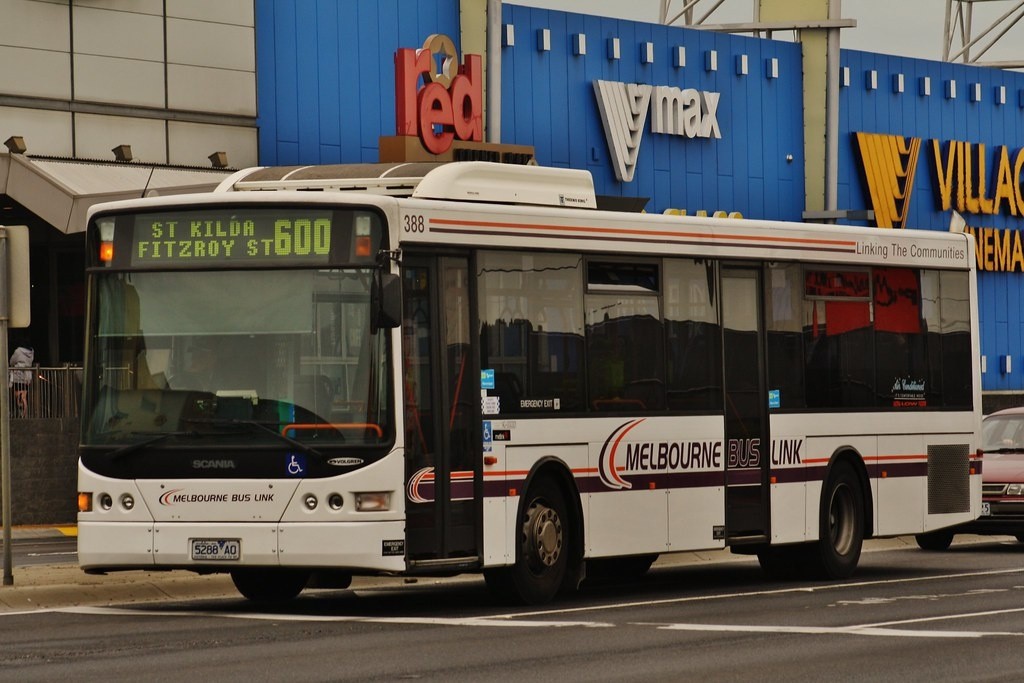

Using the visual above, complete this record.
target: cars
[915,407,1024,552]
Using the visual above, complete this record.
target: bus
[77,161,983,614]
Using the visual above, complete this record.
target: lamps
[207,151,229,167]
[111,144,133,161]
[4,135,26,154]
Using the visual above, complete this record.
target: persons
[10,334,34,417]
[1003,429,1023,448]
[171,336,234,390]
